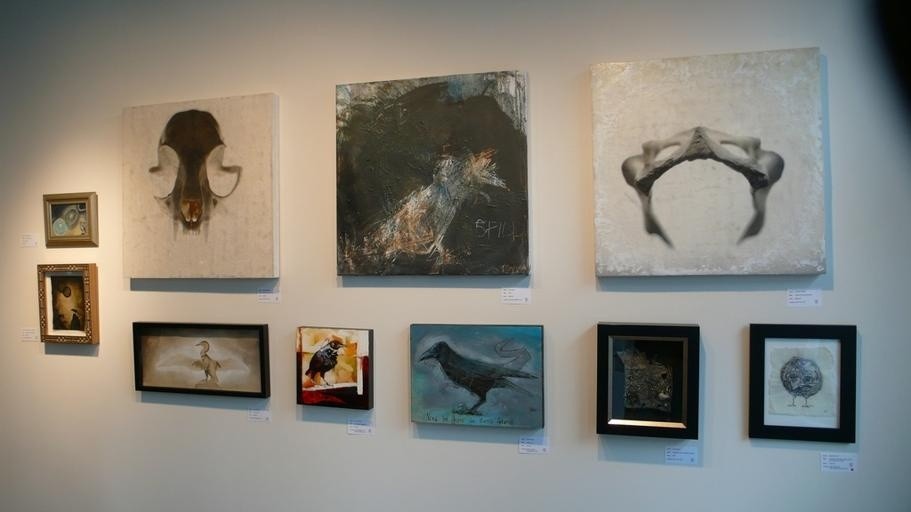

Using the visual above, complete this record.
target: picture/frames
[748,323,857,443]
[43,193,99,248]
[596,322,700,439]
[37,264,99,345]
[132,322,270,398]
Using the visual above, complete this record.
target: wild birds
[420,341,539,415]
[305,340,347,388]
[192,341,221,384]
